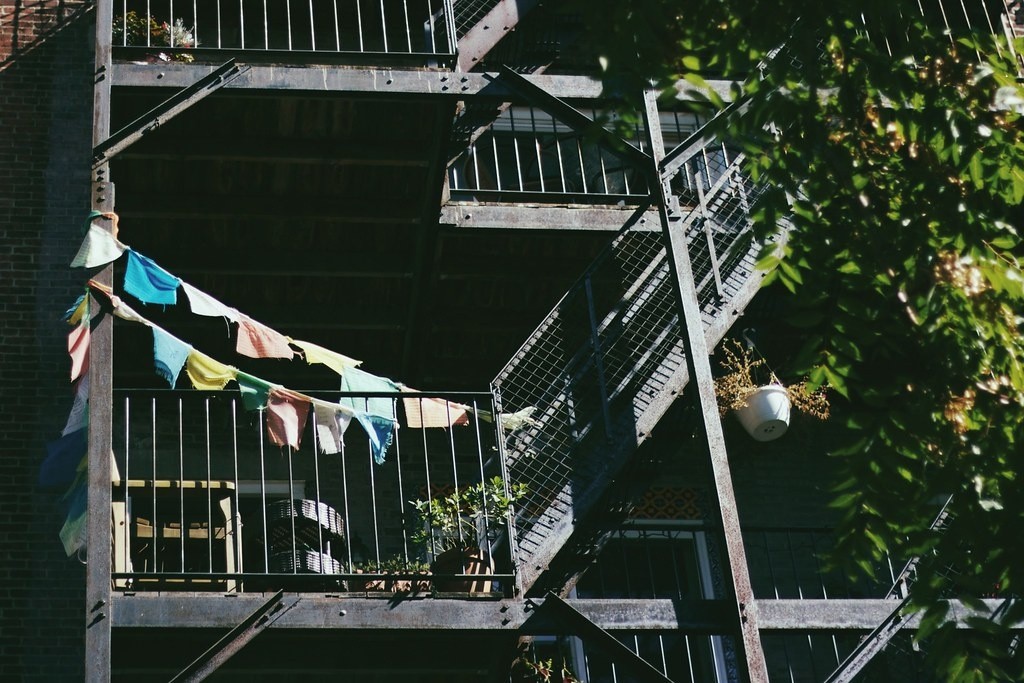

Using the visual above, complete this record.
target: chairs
[110,444,240,592]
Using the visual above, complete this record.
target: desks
[252,502,348,593]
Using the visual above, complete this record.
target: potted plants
[411,561,433,591]
[409,477,528,591]
[389,554,411,592]
[345,559,389,590]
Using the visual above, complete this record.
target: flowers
[712,337,832,422]
[112,12,200,63]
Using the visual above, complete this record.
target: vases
[132,48,171,63]
[735,385,791,442]
